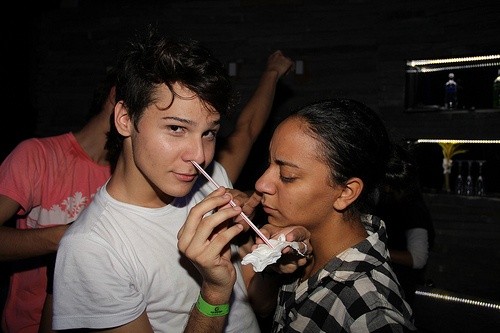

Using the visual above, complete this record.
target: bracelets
[196,293,230,317]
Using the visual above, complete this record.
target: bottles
[444,72,457,110]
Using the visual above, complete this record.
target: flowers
[438,141,467,166]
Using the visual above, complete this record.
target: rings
[301,242,308,256]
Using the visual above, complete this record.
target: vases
[441,158,453,190]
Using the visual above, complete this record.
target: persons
[212,49,414,333]
[53,27,313,333]
[0,83,117,333]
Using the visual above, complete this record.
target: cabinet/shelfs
[401,49,500,299]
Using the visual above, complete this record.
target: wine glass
[455,159,486,197]
